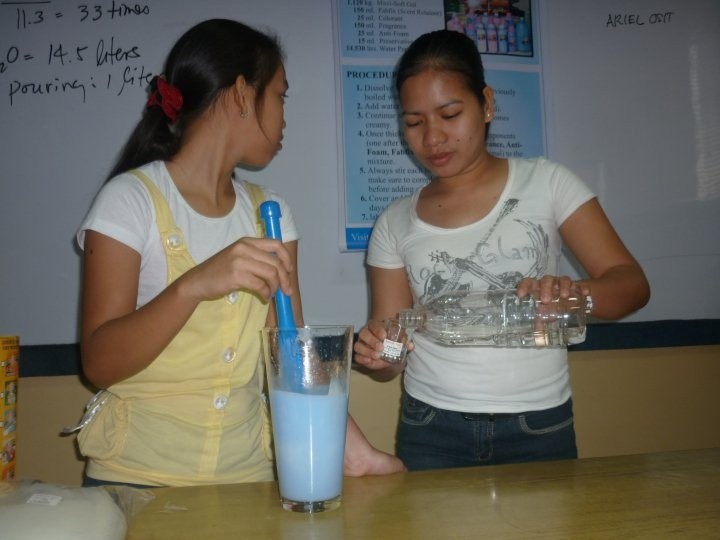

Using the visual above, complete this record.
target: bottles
[397,289,588,348]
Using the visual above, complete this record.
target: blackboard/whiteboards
[0,0,720,377]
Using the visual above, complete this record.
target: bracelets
[582,294,593,316]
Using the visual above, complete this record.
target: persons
[353,29,651,471]
[77,19,406,489]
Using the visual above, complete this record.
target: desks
[123,442,720,540]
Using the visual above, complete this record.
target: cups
[382,317,416,365]
[261,324,354,514]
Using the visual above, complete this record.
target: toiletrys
[446,3,532,54]
